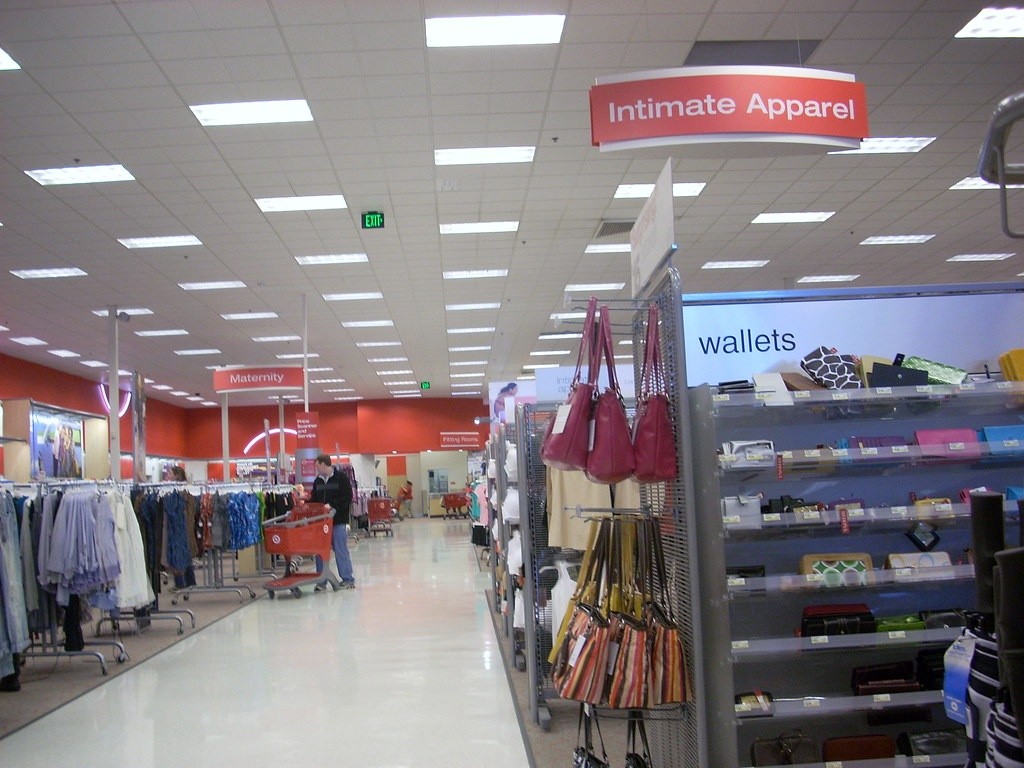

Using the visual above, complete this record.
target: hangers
[0,476,295,502]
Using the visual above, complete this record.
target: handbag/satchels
[717,420,1024,473]
[539,298,599,471]
[573,701,610,768]
[793,604,975,697]
[548,515,693,710]
[624,709,653,768]
[880,552,952,592]
[726,565,767,596]
[751,730,819,766]
[632,303,676,483]
[720,482,998,535]
[822,734,896,762]
[735,692,774,718]
[585,305,636,484]
[798,552,873,594]
[903,724,967,756]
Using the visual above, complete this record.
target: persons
[300,454,355,592]
[396,481,415,518]
[53,423,79,478]
[494,383,519,418]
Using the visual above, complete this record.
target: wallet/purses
[717,347,968,420]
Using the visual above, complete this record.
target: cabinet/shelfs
[683,363,1024,768]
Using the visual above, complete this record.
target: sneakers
[314,584,326,591]
[338,581,355,590]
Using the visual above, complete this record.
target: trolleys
[366,496,396,537]
[261,502,344,600]
[439,491,469,521]
